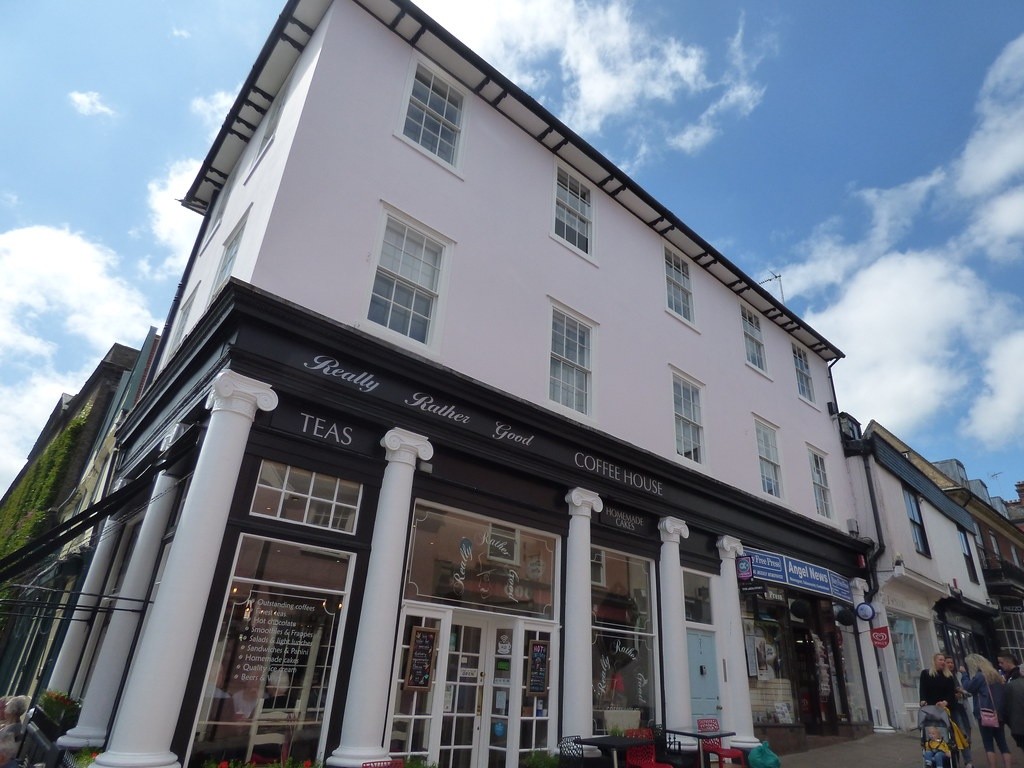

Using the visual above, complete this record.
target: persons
[925,726,950,768]
[0,694,31,742]
[1001,662,1024,755]
[947,658,976,768]
[919,651,957,712]
[0,696,15,723]
[960,654,1013,768]
[997,654,1024,729]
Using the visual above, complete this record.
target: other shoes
[964,763,974,768]
[926,762,931,766]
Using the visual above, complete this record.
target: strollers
[918,702,959,768]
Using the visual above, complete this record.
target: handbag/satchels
[979,708,999,728]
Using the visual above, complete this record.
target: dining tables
[574,734,652,768]
[662,727,736,768]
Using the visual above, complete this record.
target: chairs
[696,718,746,768]
[624,727,673,768]
[558,736,584,768]
